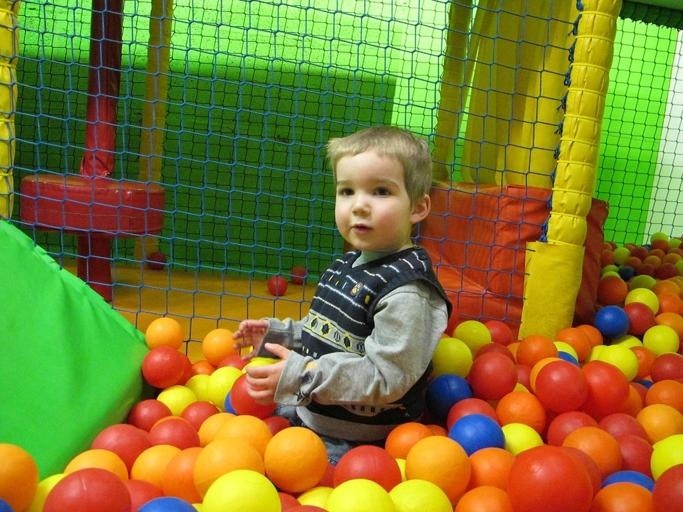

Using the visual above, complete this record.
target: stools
[17,173,169,302]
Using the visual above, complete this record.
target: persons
[233,128,452,467]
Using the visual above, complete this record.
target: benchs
[421,180,604,339]
